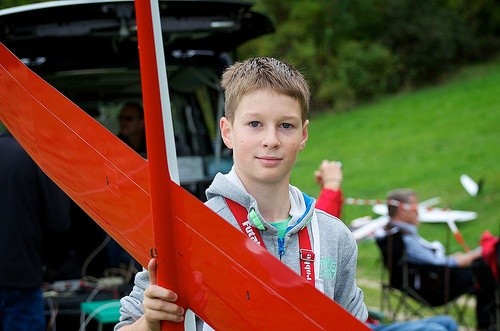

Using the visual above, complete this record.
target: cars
[0,0,277,316]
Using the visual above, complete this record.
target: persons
[113,55,371,331]
[113,102,189,164]
[311,157,344,220]
[374,186,484,308]
[0,120,72,331]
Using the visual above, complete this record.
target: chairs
[374,225,474,331]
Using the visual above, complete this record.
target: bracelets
[322,176,343,185]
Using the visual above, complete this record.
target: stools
[79,300,122,331]
